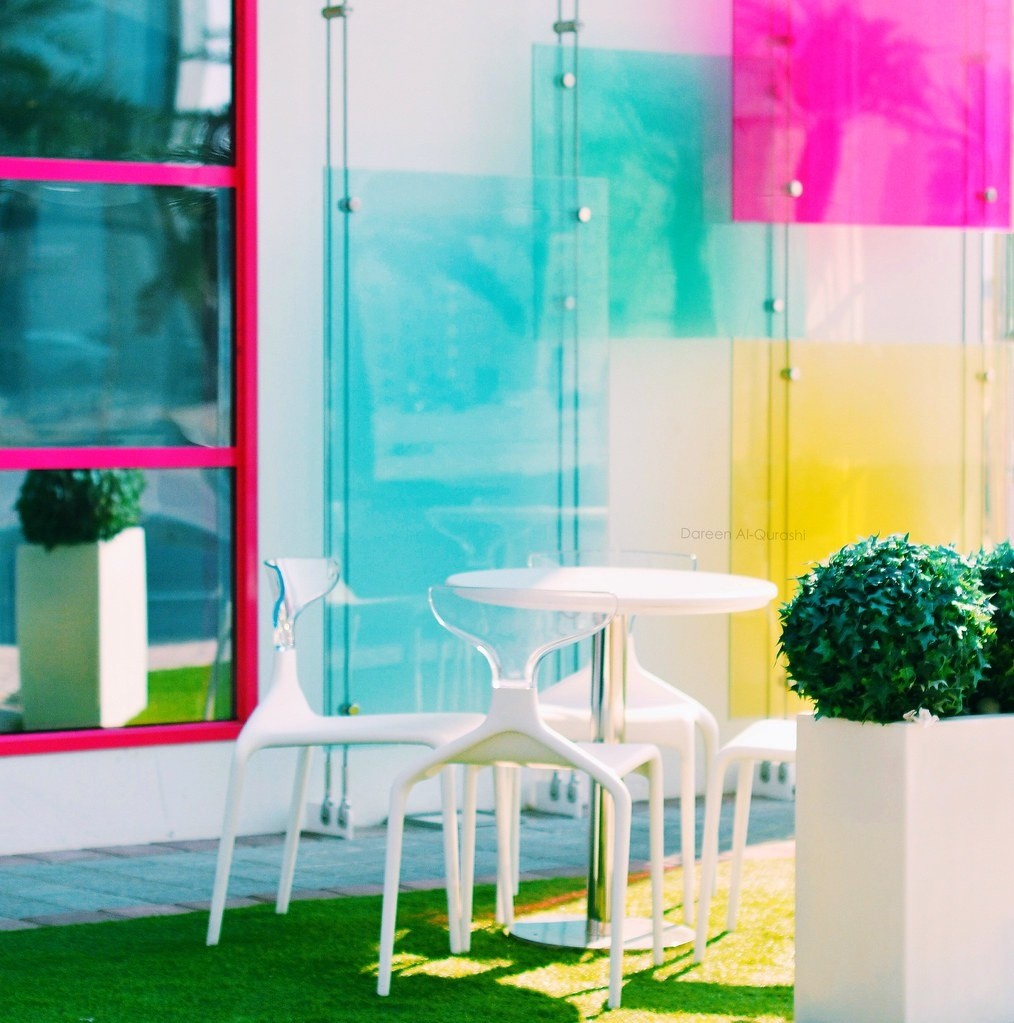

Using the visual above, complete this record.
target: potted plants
[778,545,1014,1023]
[19,473,149,730]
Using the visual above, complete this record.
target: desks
[451,561,782,953]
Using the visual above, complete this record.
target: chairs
[208,555,797,1008]
